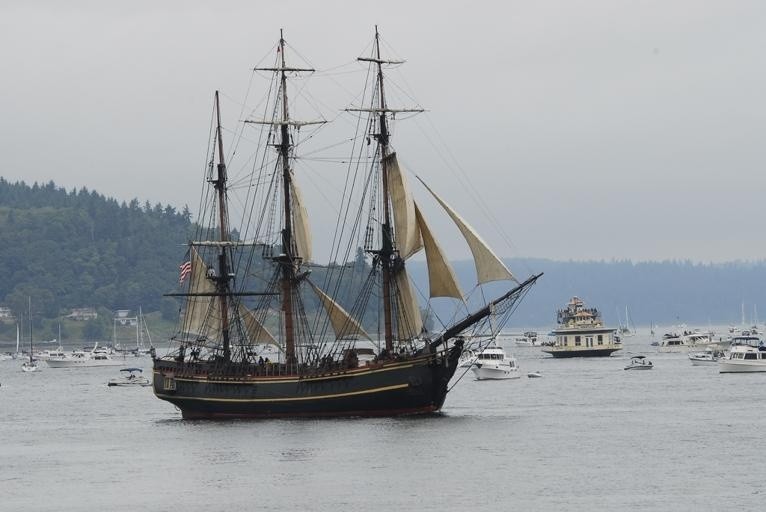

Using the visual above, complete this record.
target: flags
[179,261,191,288]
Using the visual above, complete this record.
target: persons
[327,354,332,363]
[399,347,403,354]
[322,355,327,363]
[265,357,271,366]
[259,356,264,366]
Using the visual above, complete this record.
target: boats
[546,296,623,359]
[107,368,151,387]
[462,344,522,380]
[626,354,654,371]
[515,327,544,346]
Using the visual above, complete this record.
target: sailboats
[621,303,637,338]
[152,24,544,418]
[650,295,765,374]
[0,297,153,371]
